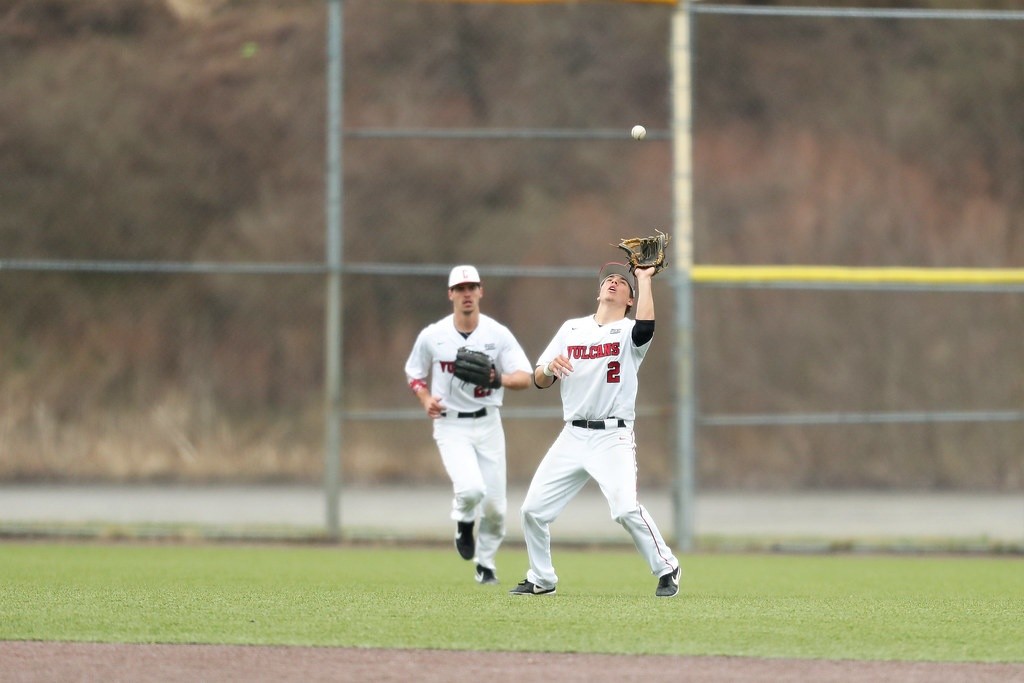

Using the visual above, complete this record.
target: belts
[441,408,486,419]
[572,419,626,430]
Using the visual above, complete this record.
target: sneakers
[509,578,556,596]
[473,564,500,585]
[454,518,476,560]
[655,562,681,597]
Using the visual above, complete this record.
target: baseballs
[630,124,648,143]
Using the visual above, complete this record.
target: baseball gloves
[619,233,668,277]
[453,348,503,391]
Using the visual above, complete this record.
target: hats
[599,261,635,297]
[447,265,480,288]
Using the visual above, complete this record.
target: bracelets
[543,360,554,376]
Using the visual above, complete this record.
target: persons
[509,239,684,600]
[401,263,534,585]
